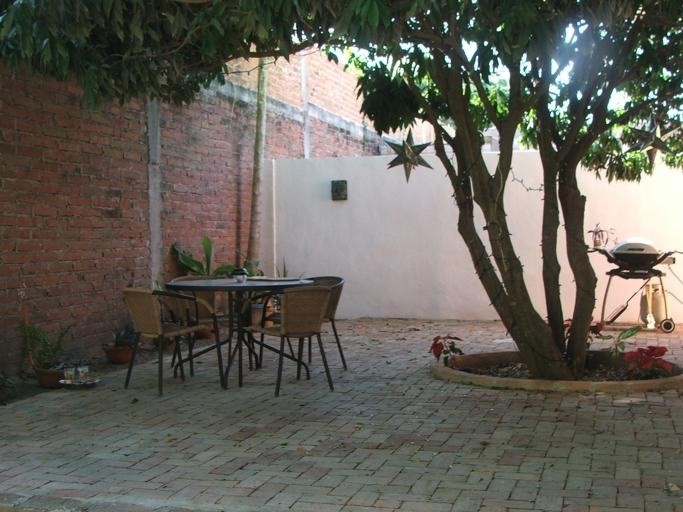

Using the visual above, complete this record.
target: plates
[58,376,105,389]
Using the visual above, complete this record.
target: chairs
[120,271,350,398]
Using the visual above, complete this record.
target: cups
[77,366,88,383]
[63,367,74,384]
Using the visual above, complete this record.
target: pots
[590,241,683,267]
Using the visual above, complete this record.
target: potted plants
[106,324,138,364]
[18,323,73,387]
